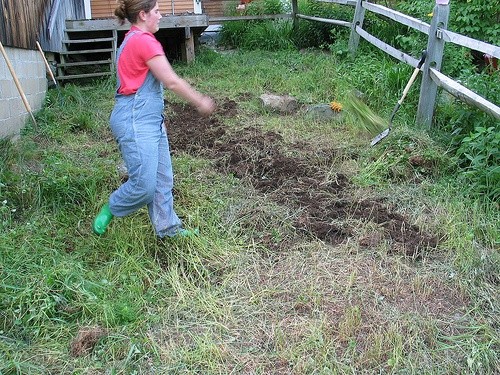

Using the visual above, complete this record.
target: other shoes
[92,203,114,235]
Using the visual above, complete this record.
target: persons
[94,0,220,240]
